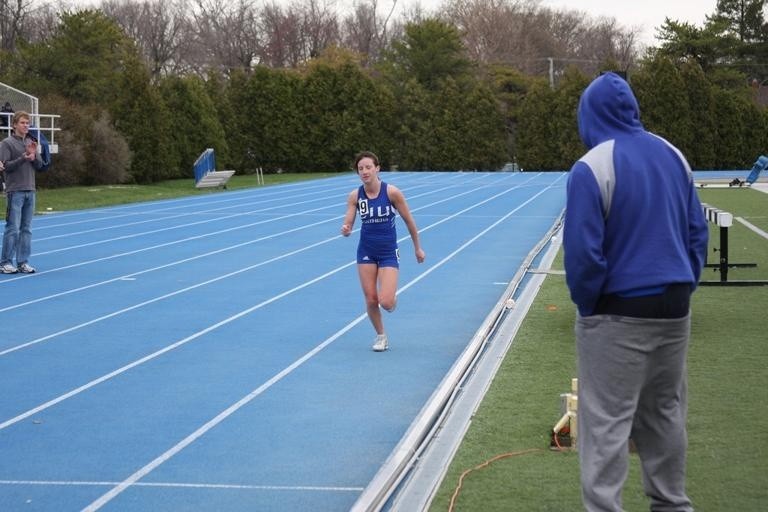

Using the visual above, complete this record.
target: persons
[560,66,712,512]
[339,149,425,355]
[0,101,15,133]
[0,108,39,275]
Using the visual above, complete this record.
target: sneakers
[16,263,35,273]
[372,338,388,351]
[0,264,18,274]
